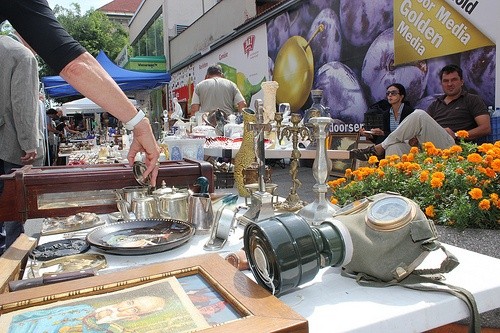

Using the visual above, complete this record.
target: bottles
[232,105,263,198]
[243,214,356,298]
[304,89,334,151]
[486,106,500,117]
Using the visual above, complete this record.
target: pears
[272,24,324,112]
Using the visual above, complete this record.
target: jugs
[113,161,190,222]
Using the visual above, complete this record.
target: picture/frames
[325,132,360,177]
[0,254,310,333]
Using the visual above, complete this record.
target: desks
[22,192,500,333]
[57,140,350,162]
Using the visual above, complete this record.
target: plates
[86,219,196,256]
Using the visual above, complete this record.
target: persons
[190,63,249,123]
[349,64,493,163]
[0,26,40,265]
[359,82,414,146]
[1,1,162,187]
[28,76,132,166]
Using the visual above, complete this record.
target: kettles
[190,193,214,231]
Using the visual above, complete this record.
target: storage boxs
[476,116,500,142]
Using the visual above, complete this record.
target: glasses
[386,90,402,96]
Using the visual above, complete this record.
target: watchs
[454,132,459,140]
[120,107,146,132]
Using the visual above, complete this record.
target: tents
[52,95,136,119]
[42,49,172,104]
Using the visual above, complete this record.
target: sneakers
[350,145,379,161]
